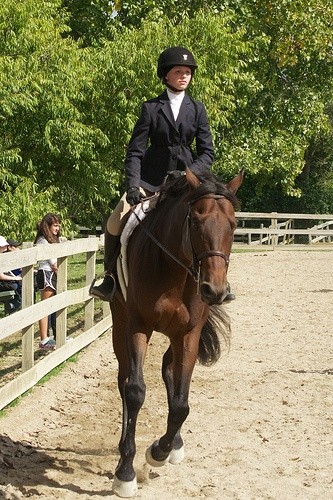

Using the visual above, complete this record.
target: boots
[91,227,121,301]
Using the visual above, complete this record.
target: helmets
[157,47,198,79]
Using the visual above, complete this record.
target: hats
[0,236,10,247]
[7,239,22,247]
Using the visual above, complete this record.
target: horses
[109,162,247,498]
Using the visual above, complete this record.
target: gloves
[126,187,146,206]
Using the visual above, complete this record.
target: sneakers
[66,337,73,342]
[39,337,56,350]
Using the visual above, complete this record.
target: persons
[92,46,237,302]
[0,235,22,316]
[33,212,61,350]
[3,238,39,305]
